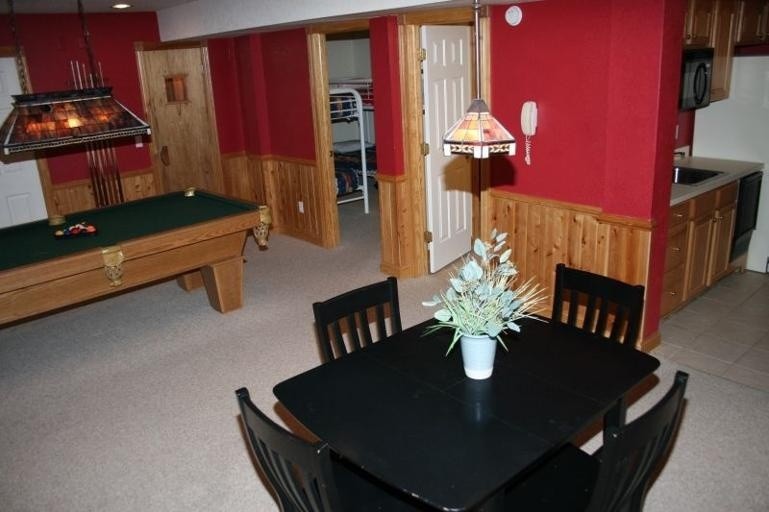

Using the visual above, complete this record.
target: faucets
[673,152,685,159]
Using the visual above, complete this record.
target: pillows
[329,139,374,158]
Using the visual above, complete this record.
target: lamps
[1,0,154,164]
[438,0,525,162]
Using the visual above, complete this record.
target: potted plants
[417,224,556,383]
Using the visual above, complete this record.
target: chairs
[232,385,426,510]
[495,368,692,512]
[308,272,404,366]
[545,257,651,445]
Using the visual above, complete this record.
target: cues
[69,60,126,209]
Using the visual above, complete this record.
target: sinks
[672,166,724,186]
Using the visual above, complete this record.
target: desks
[269,304,662,512]
[1,184,274,329]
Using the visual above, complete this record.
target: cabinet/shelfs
[682,1,714,50]
[707,177,740,293]
[661,197,690,323]
[709,1,740,109]
[682,186,720,316]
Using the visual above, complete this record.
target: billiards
[55,221,97,238]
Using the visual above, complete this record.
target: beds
[329,76,378,188]
[327,86,372,219]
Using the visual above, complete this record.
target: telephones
[520,101,537,136]
